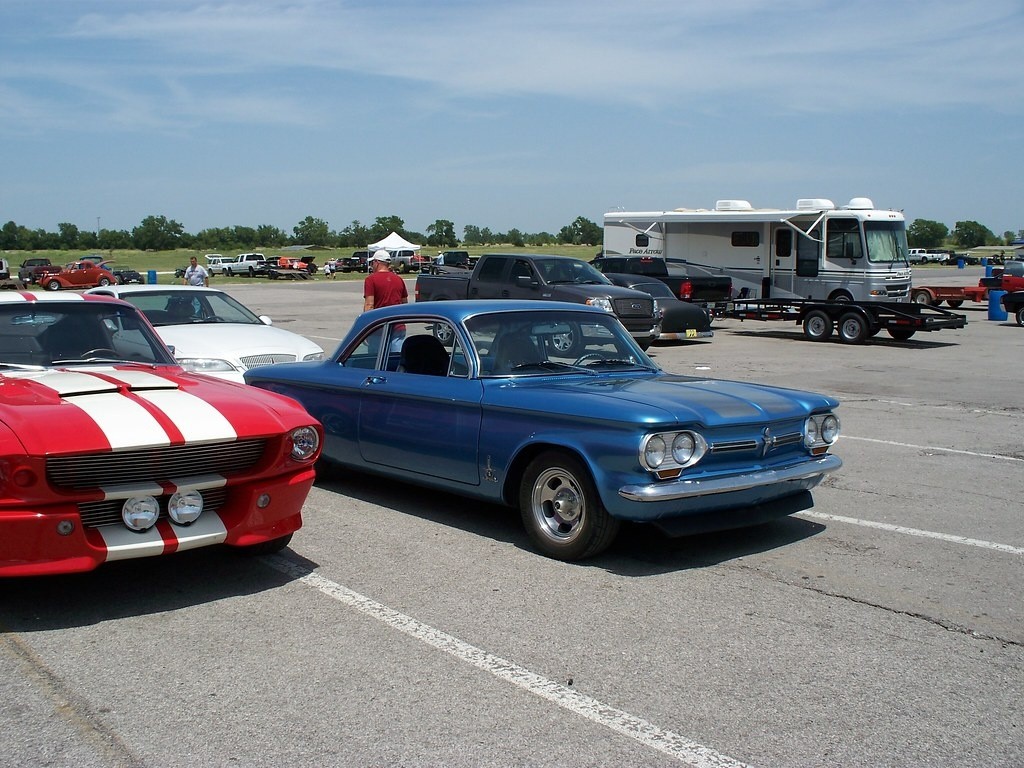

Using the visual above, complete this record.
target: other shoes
[333,275,336,279]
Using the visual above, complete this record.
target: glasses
[371,260,374,265]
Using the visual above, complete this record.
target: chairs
[493,333,543,371]
[548,265,568,280]
[168,303,195,322]
[846,242,853,257]
[396,335,468,375]
[510,265,533,282]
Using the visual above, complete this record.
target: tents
[367,231,422,274]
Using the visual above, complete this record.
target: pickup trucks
[415,254,663,360]
[575,256,733,318]
[66,256,113,275]
[335,249,481,276]
[17,258,63,285]
[907,248,942,265]
[228,253,312,279]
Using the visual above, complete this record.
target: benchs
[140,310,168,323]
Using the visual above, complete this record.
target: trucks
[909,260,1024,308]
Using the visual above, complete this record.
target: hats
[330,258,335,260]
[437,251,441,254]
[367,250,392,263]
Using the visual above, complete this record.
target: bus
[602,197,914,307]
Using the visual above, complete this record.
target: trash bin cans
[981,258,987,266]
[958,260,964,269]
[148,271,156,284]
[986,266,992,276]
[988,290,1008,321]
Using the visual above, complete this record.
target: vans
[205,253,234,276]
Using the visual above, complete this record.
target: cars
[241,299,843,562]
[82,285,325,384]
[596,272,714,341]
[40,260,145,291]
[1,290,325,579]
[1003,290,1024,326]
[947,254,979,266]
[0,258,12,279]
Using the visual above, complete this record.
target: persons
[183,256,210,316]
[397,261,405,273]
[436,250,445,275]
[323,261,331,278]
[328,257,337,279]
[363,249,409,355]
[288,260,300,270]
